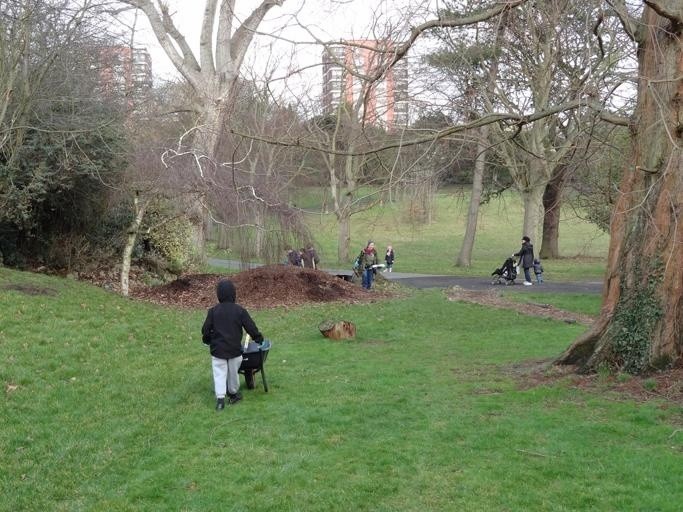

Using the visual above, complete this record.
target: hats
[521,236,530,242]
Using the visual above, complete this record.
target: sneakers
[216,397,225,411]
[523,280,533,286]
[229,393,242,405]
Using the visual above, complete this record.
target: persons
[306,243,320,269]
[511,235,535,286]
[284,245,299,266]
[200,278,266,413]
[352,238,382,290]
[382,246,395,273]
[532,258,545,284]
[298,248,317,270]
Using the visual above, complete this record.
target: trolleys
[491,256,518,286]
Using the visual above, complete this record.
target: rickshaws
[236,339,271,395]
